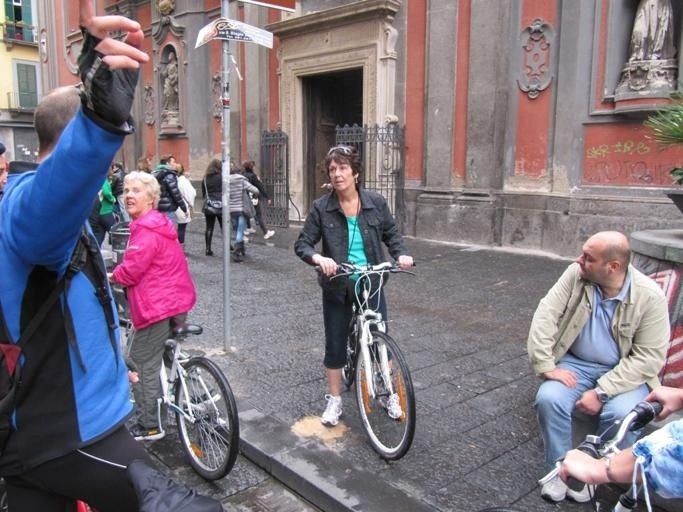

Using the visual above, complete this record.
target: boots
[204,228,214,255]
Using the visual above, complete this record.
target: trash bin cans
[109,222,131,271]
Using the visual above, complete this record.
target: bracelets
[605,457,617,483]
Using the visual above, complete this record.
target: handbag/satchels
[204,198,221,214]
[321,273,348,305]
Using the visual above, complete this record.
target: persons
[555,386,683,500]
[2,16,151,511]
[1,144,197,511]
[201,159,222,256]
[527,231,671,504]
[230,161,235,171]
[242,161,275,240]
[230,168,260,261]
[294,145,414,426]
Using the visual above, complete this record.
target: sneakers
[379,393,404,421]
[263,229,274,238]
[540,472,596,502]
[242,226,256,242]
[182,390,221,407]
[229,242,244,263]
[320,395,344,427]
[128,424,164,441]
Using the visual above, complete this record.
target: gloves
[74,24,140,127]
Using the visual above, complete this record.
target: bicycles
[107,272,241,483]
[314,260,417,460]
[538,401,683,512]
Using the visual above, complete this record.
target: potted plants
[644,78,683,220]
